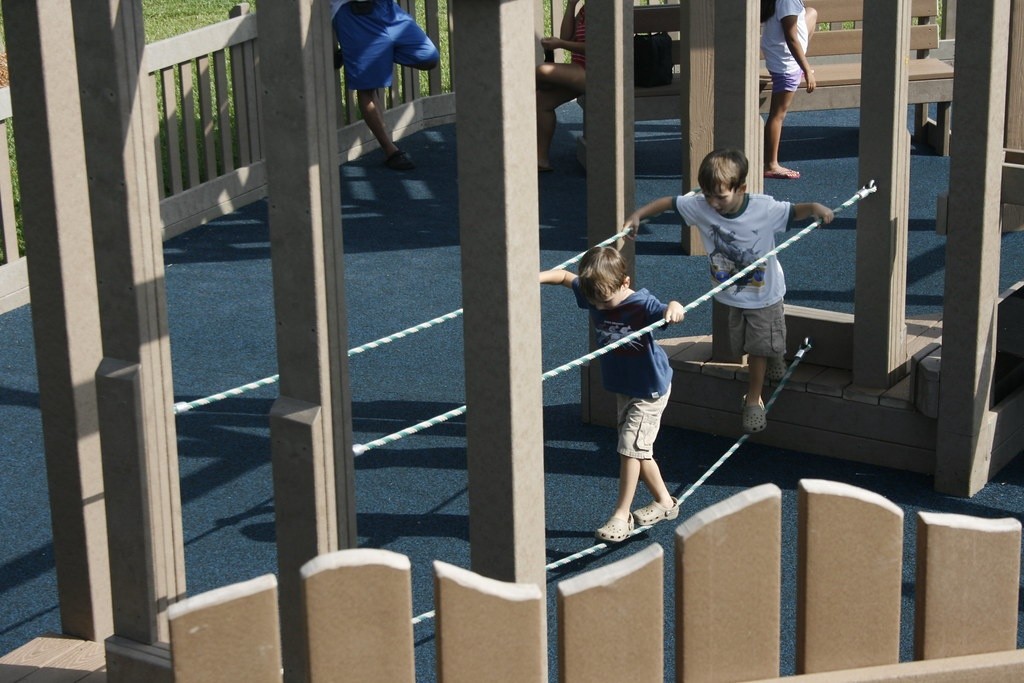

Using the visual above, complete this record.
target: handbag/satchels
[634,31,673,87]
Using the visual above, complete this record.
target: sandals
[741,393,766,433]
[595,512,634,542]
[633,497,679,525]
[766,356,787,380]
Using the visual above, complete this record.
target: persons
[538,0,586,170]
[621,148,834,432]
[539,245,685,542]
[329,0,438,171]
[760,0,818,180]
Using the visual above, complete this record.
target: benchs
[576,0,954,161]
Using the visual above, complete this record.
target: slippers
[764,167,800,178]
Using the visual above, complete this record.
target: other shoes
[335,48,343,69]
[387,149,416,169]
